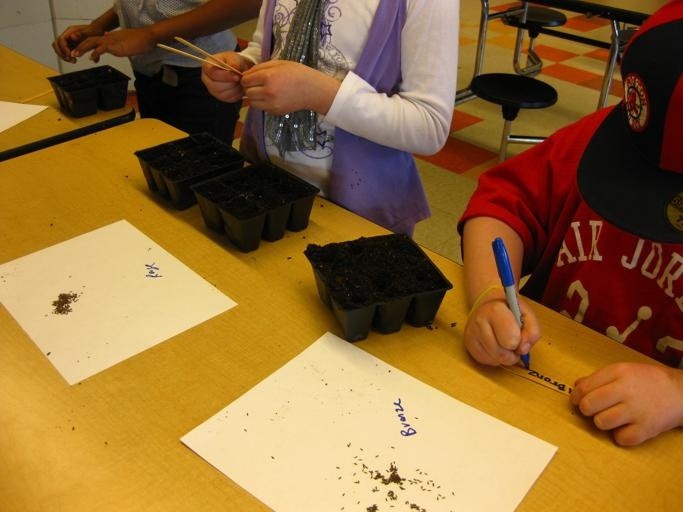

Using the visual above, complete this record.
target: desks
[450,1,661,165]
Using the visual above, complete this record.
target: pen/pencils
[492,238,529,370]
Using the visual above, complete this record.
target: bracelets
[467,285,521,318]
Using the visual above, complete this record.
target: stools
[501,6,567,77]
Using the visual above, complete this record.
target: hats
[576,0,683,246]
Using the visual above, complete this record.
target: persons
[199,0,458,240]
[53,0,263,147]
[454,0,682,447]
